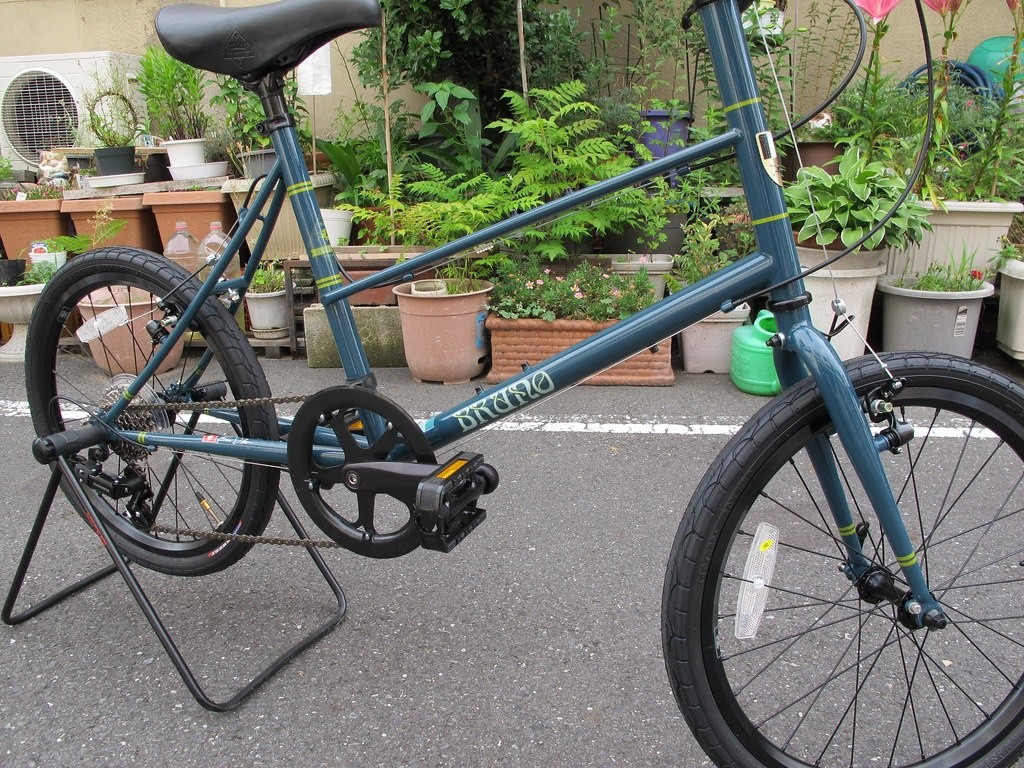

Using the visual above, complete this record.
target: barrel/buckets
[730,309,782,397]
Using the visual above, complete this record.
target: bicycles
[0,0,1024,768]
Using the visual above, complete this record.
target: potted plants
[0,145,186,377]
[87,1,1024,394]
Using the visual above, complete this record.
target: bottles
[196,221,247,335]
[163,222,203,344]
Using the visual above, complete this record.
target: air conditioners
[1,51,151,172]
